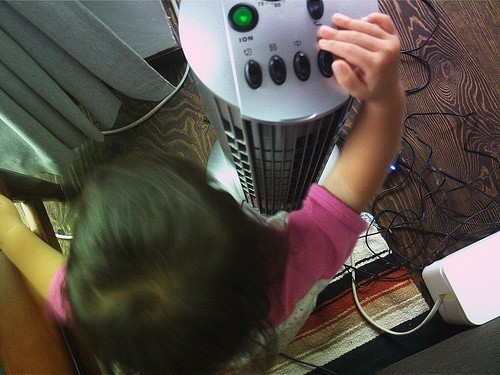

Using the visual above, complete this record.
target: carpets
[264,211,430,375]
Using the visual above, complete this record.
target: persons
[0,12,407,375]
